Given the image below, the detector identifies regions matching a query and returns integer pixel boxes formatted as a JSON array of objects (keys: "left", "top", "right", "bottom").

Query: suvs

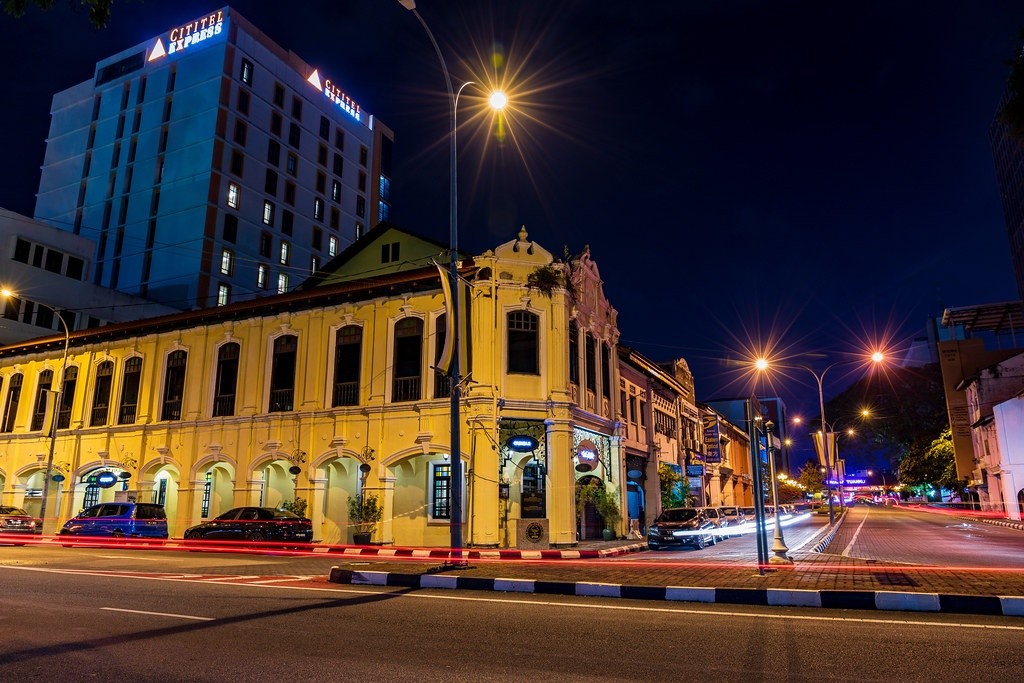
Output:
[{"left": 647, "top": 507, "right": 717, "bottom": 551}]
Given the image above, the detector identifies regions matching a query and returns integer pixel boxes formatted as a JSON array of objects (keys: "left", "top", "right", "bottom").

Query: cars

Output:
[
  {"left": 697, "top": 506, "right": 731, "bottom": 541},
  {"left": 0, "top": 505, "right": 36, "bottom": 546},
  {"left": 183, "top": 506, "right": 313, "bottom": 554}
]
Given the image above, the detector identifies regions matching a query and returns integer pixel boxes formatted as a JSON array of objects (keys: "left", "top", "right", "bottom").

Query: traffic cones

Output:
[{"left": 741, "top": 503, "right": 798, "bottom": 525}]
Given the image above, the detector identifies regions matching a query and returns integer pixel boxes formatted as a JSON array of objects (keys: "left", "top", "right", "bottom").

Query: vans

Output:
[
  {"left": 718, "top": 505, "right": 749, "bottom": 537},
  {"left": 56, "top": 501, "right": 169, "bottom": 549}
]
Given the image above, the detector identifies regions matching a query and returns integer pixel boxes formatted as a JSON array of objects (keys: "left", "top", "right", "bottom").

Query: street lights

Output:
[
  {"left": 834, "top": 427, "right": 855, "bottom": 514},
  {"left": 793, "top": 409, "right": 870, "bottom": 432},
  {"left": 0, "top": 287, "right": 69, "bottom": 518},
  {"left": 783, "top": 437, "right": 791, "bottom": 476},
  {"left": 753, "top": 347, "right": 885, "bottom": 526},
  {"left": 400, "top": 0, "right": 511, "bottom": 556}
]
